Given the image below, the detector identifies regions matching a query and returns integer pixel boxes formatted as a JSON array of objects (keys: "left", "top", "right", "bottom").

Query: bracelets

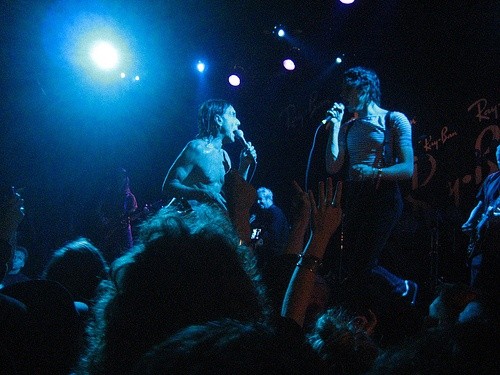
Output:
[
  {"left": 296, "top": 253, "right": 323, "bottom": 274},
  {"left": 372, "top": 166, "right": 375, "bottom": 177},
  {"left": 377, "top": 167, "right": 383, "bottom": 179}
]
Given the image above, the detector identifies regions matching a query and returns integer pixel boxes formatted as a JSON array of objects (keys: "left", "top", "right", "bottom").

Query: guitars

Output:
[
  {"left": 85, "top": 201, "right": 151, "bottom": 244},
  {"left": 467, "top": 195, "right": 500, "bottom": 259}
]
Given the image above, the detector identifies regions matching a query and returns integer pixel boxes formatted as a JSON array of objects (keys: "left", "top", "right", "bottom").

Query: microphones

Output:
[
  {"left": 235, "top": 130, "right": 257, "bottom": 164},
  {"left": 322, "top": 102, "right": 345, "bottom": 125}
]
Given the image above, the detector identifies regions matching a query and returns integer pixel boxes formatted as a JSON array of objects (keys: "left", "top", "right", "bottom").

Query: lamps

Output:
[
  {"left": 282, "top": 46, "right": 301, "bottom": 70},
  {"left": 228, "top": 63, "right": 249, "bottom": 87},
  {"left": 272, "top": 23, "right": 288, "bottom": 40}
]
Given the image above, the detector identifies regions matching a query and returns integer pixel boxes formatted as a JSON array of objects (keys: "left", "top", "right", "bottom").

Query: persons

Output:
[
  {"left": 161, "top": 97, "right": 259, "bottom": 216},
  {"left": 97, "top": 168, "right": 138, "bottom": 263},
  {"left": 252, "top": 186, "right": 290, "bottom": 257},
  {"left": 460, "top": 145, "right": 500, "bottom": 290},
  {"left": 323, "top": 65, "right": 414, "bottom": 265},
  {"left": 0, "top": 178, "right": 500, "bottom": 375}
]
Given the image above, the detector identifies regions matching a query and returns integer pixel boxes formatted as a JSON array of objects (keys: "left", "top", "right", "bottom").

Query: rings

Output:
[
  {"left": 331, "top": 201, "right": 341, "bottom": 208},
  {"left": 325, "top": 199, "right": 330, "bottom": 206}
]
